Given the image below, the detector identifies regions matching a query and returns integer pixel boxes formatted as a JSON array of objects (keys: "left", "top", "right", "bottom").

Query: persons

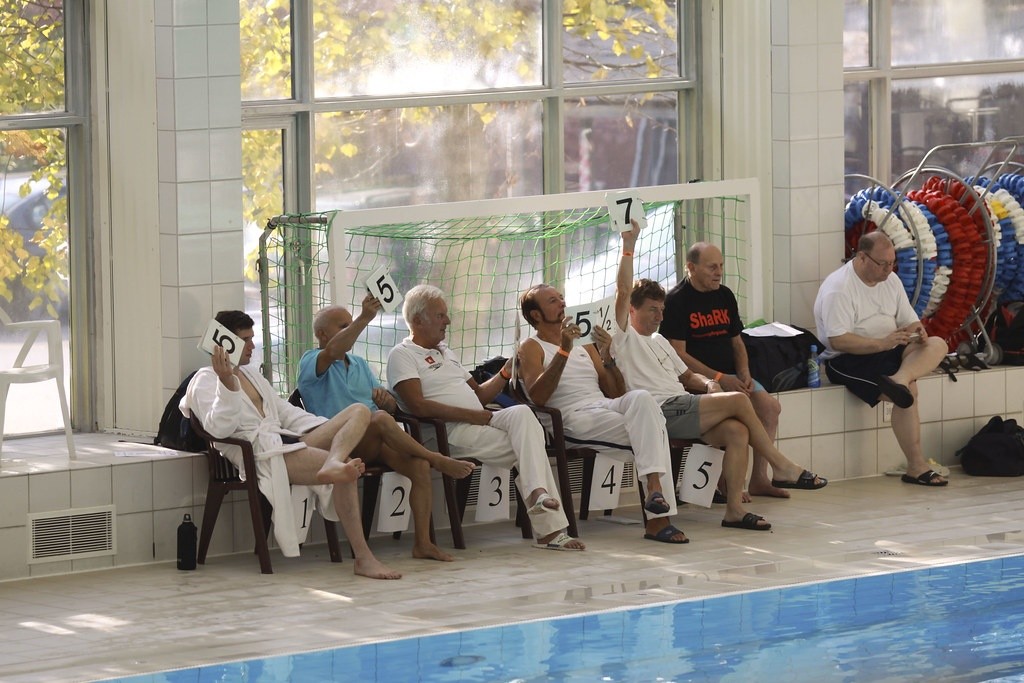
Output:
[
  {"left": 188, "top": 310, "right": 403, "bottom": 580},
  {"left": 659, "top": 242, "right": 791, "bottom": 503},
  {"left": 386, "top": 285, "right": 586, "bottom": 551},
  {"left": 608, "top": 219, "right": 827, "bottom": 531},
  {"left": 297, "top": 292, "right": 476, "bottom": 562},
  {"left": 518, "top": 284, "right": 689, "bottom": 544},
  {"left": 814, "top": 231, "right": 948, "bottom": 485}
]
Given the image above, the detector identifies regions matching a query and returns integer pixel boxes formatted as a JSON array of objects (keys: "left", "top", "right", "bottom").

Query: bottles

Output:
[
  {"left": 807, "top": 345, "right": 820, "bottom": 388},
  {"left": 177, "top": 514, "right": 197, "bottom": 570}
]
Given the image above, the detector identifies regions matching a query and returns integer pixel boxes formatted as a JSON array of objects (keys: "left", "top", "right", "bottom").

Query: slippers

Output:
[
  {"left": 772, "top": 469, "right": 828, "bottom": 490},
  {"left": 721, "top": 512, "right": 771, "bottom": 531},
  {"left": 878, "top": 376, "right": 914, "bottom": 408},
  {"left": 644, "top": 491, "right": 670, "bottom": 515},
  {"left": 901, "top": 470, "right": 948, "bottom": 487},
  {"left": 527, "top": 492, "right": 560, "bottom": 515},
  {"left": 710, "top": 482, "right": 728, "bottom": 504},
  {"left": 643, "top": 523, "right": 689, "bottom": 544},
  {"left": 532, "top": 532, "right": 583, "bottom": 551}
]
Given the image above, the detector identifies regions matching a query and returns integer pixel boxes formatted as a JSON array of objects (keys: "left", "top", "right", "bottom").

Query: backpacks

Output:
[{"left": 154, "top": 370, "right": 209, "bottom": 453}]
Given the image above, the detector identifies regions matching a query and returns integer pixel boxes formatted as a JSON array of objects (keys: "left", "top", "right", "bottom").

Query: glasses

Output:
[{"left": 863, "top": 251, "right": 896, "bottom": 270}]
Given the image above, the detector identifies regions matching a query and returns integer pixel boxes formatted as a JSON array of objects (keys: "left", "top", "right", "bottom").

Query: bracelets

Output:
[
  {"left": 715, "top": 372, "right": 722, "bottom": 380},
  {"left": 623, "top": 250, "right": 634, "bottom": 256},
  {"left": 557, "top": 345, "right": 569, "bottom": 358},
  {"left": 500, "top": 366, "right": 510, "bottom": 380},
  {"left": 705, "top": 380, "right": 717, "bottom": 387},
  {"left": 603, "top": 357, "right": 616, "bottom": 369}
]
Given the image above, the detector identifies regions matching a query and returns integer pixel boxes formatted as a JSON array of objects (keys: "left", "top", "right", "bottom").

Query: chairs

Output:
[
  {"left": 0, "top": 307, "right": 76, "bottom": 457},
  {"left": 188, "top": 407, "right": 343, "bottom": 574},
  {"left": 508, "top": 379, "right": 648, "bottom": 538},
  {"left": 670, "top": 437, "right": 727, "bottom": 506},
  {"left": 385, "top": 389, "right": 534, "bottom": 549},
  {"left": 299, "top": 396, "right": 437, "bottom": 560}
]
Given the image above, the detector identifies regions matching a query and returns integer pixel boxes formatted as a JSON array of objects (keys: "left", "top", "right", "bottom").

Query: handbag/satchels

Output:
[
  {"left": 468, "top": 354, "right": 525, "bottom": 405},
  {"left": 743, "top": 321, "right": 827, "bottom": 392},
  {"left": 956, "top": 416, "right": 1024, "bottom": 477}
]
